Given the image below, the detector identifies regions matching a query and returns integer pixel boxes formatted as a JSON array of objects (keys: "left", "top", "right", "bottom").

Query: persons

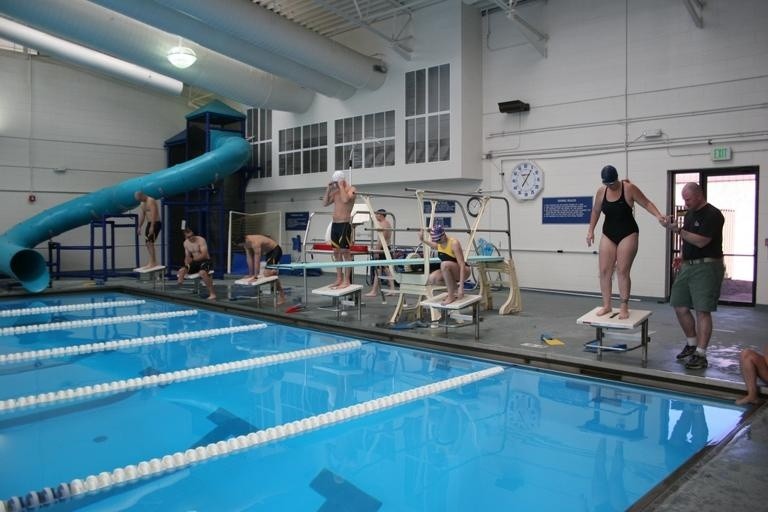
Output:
[
  {"left": 363, "top": 210, "right": 395, "bottom": 297},
  {"left": 417, "top": 225, "right": 471, "bottom": 305},
  {"left": 232, "top": 233, "right": 289, "bottom": 306},
  {"left": 175, "top": 228, "right": 216, "bottom": 300},
  {"left": 134, "top": 191, "right": 165, "bottom": 269},
  {"left": 732, "top": 347, "right": 768, "bottom": 406},
  {"left": 321, "top": 171, "right": 357, "bottom": 290},
  {"left": 127, "top": 294, "right": 711, "bottom": 511},
  {"left": 585, "top": 165, "right": 675, "bottom": 321},
  {"left": 659, "top": 182, "right": 727, "bottom": 369}
]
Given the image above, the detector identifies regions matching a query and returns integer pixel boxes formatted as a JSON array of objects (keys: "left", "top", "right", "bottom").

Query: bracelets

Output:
[{"left": 677, "top": 227, "right": 683, "bottom": 235}]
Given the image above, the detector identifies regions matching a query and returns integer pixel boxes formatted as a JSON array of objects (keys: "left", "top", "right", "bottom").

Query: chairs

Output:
[{"left": 326, "top": 201, "right": 371, "bottom": 246}]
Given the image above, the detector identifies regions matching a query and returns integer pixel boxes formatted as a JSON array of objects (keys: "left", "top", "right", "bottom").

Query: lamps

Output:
[{"left": 166, "top": 31, "right": 200, "bottom": 70}]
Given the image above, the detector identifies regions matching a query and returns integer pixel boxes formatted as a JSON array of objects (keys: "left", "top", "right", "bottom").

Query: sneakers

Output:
[
  {"left": 676, "top": 344, "right": 697, "bottom": 359},
  {"left": 685, "top": 352, "right": 708, "bottom": 370}
]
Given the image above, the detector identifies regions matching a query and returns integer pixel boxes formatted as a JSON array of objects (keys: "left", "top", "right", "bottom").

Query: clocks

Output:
[{"left": 507, "top": 160, "right": 546, "bottom": 202}]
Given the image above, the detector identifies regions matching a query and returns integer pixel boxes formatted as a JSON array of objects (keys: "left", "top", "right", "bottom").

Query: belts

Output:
[{"left": 686, "top": 258, "right": 721, "bottom": 265}]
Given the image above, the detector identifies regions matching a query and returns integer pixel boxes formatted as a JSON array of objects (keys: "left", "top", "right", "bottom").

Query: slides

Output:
[{"left": 0, "top": 129, "right": 251, "bottom": 294}]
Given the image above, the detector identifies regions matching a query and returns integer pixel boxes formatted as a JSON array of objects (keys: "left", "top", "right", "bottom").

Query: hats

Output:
[
  {"left": 430, "top": 225, "right": 445, "bottom": 242},
  {"left": 601, "top": 165, "right": 618, "bottom": 182},
  {"left": 332, "top": 171, "right": 346, "bottom": 182}
]
